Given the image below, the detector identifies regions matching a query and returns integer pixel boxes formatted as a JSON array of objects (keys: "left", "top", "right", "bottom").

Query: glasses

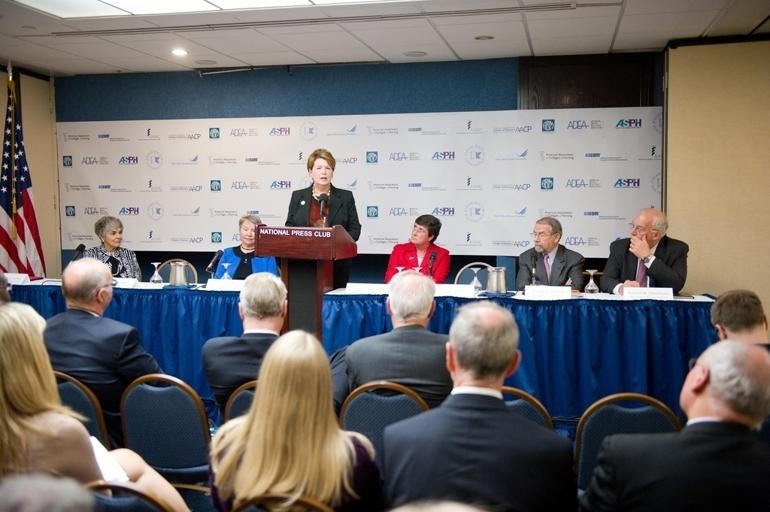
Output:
[
  {"left": 104, "top": 279, "right": 117, "bottom": 288},
  {"left": 530, "top": 232, "right": 553, "bottom": 238}
]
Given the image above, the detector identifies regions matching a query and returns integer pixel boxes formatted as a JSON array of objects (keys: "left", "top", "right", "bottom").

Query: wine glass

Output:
[
  {"left": 220, "top": 263, "right": 233, "bottom": 280},
  {"left": 584, "top": 269, "right": 600, "bottom": 294},
  {"left": 394, "top": 267, "right": 423, "bottom": 273},
  {"left": 469, "top": 267, "right": 482, "bottom": 292},
  {"left": 149, "top": 263, "right": 164, "bottom": 287}
]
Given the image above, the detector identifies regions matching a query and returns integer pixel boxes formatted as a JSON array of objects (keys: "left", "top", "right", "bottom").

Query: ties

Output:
[
  {"left": 543, "top": 253, "right": 551, "bottom": 283},
  {"left": 636, "top": 258, "right": 646, "bottom": 288}
]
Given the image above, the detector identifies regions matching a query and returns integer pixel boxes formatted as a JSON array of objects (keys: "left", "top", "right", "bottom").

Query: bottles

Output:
[
  {"left": 170, "top": 261, "right": 190, "bottom": 287},
  {"left": 486, "top": 267, "right": 507, "bottom": 293}
]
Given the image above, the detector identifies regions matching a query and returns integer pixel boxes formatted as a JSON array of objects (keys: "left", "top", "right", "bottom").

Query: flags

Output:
[{"left": 0, "top": 67, "right": 47, "bottom": 282}]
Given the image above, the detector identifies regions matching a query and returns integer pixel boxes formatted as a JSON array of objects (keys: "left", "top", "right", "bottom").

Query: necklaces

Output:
[
  {"left": 311, "top": 186, "right": 332, "bottom": 201},
  {"left": 240, "top": 244, "right": 256, "bottom": 251}
]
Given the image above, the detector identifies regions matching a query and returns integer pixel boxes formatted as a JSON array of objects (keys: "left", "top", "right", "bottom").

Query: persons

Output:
[
  {"left": 79, "top": 217, "right": 142, "bottom": 282},
  {"left": 213, "top": 215, "right": 281, "bottom": 278},
  {"left": 374, "top": 300, "right": 578, "bottom": 512},
  {"left": 1, "top": 258, "right": 451, "bottom": 512},
  {"left": 577, "top": 338, "right": 768, "bottom": 512},
  {"left": 710, "top": 289, "right": 769, "bottom": 351},
  {"left": 284, "top": 149, "right": 361, "bottom": 289},
  {"left": 515, "top": 217, "right": 586, "bottom": 293},
  {"left": 384, "top": 215, "right": 450, "bottom": 284},
  {"left": 600, "top": 208, "right": 689, "bottom": 298}
]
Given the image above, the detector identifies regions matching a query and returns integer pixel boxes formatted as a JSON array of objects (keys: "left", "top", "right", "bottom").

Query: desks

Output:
[
  {"left": 9, "top": 277, "right": 243, "bottom": 428},
  {"left": 322, "top": 281, "right": 719, "bottom": 420}
]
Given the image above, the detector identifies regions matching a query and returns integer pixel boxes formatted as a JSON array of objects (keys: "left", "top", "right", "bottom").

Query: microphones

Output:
[
  {"left": 68, "top": 244, "right": 86, "bottom": 262},
  {"left": 530, "top": 255, "right": 537, "bottom": 281},
  {"left": 205, "top": 249, "right": 224, "bottom": 272},
  {"left": 319, "top": 193, "right": 330, "bottom": 226},
  {"left": 428, "top": 251, "right": 437, "bottom": 278}
]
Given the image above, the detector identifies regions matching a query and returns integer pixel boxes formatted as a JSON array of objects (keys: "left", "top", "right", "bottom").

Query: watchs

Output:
[{"left": 643, "top": 254, "right": 652, "bottom": 264}]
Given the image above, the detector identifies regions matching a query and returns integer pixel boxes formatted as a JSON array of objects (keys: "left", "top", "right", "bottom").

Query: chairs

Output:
[
  {"left": 502, "top": 385, "right": 553, "bottom": 430},
  {"left": 119, "top": 373, "right": 212, "bottom": 490},
  {"left": 230, "top": 493, "right": 334, "bottom": 512},
  {"left": 575, "top": 393, "right": 680, "bottom": 491},
  {"left": 339, "top": 379, "right": 429, "bottom": 492},
  {"left": 172, "top": 483, "right": 217, "bottom": 512},
  {"left": 225, "top": 380, "right": 259, "bottom": 423},
  {"left": 53, "top": 370, "right": 110, "bottom": 452},
  {"left": 82, "top": 480, "right": 175, "bottom": 512},
  {"left": 149, "top": 259, "right": 198, "bottom": 283},
  {"left": 454, "top": 261, "right": 493, "bottom": 289}
]
